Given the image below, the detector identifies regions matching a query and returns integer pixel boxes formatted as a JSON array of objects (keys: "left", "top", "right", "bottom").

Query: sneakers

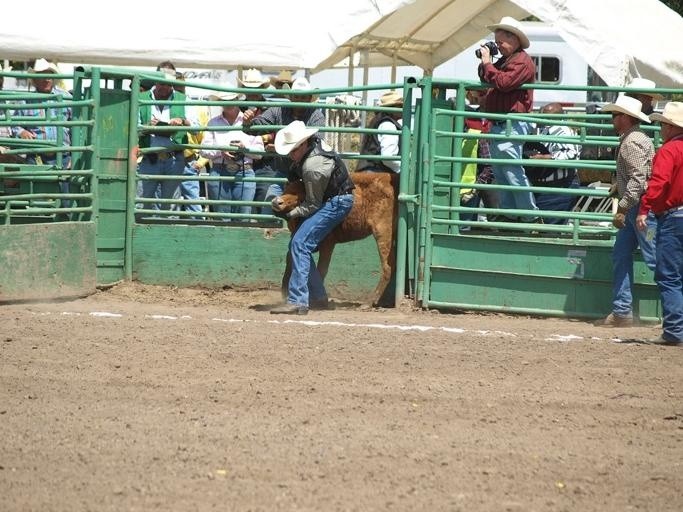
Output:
[{"left": 459, "top": 219, "right": 470, "bottom": 232}]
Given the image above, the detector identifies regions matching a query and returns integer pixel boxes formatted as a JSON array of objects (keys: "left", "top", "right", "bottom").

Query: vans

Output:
[{"left": 487, "top": 18, "right": 589, "bottom": 119}]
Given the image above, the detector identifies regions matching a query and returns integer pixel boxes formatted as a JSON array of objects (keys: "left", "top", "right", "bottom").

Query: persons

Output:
[
  {"left": 594, "top": 93, "right": 655, "bottom": 329},
  {"left": 624, "top": 78, "right": 666, "bottom": 139},
  {"left": 136, "top": 60, "right": 405, "bottom": 223},
  {"left": 478, "top": 16, "right": 545, "bottom": 234},
  {"left": 11, "top": 59, "right": 73, "bottom": 220},
  {"left": 271, "top": 120, "right": 356, "bottom": 314},
  {"left": 460, "top": 87, "right": 582, "bottom": 236},
  {"left": 636, "top": 101, "right": 683, "bottom": 346}
]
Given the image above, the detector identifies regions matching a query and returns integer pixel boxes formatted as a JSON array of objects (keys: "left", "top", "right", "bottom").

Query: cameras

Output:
[
  {"left": 475, "top": 42, "right": 498, "bottom": 58},
  {"left": 229, "top": 142, "right": 244, "bottom": 160}
]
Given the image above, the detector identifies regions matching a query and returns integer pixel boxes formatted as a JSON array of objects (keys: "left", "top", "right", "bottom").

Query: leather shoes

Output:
[
  {"left": 309, "top": 299, "right": 328, "bottom": 308},
  {"left": 270, "top": 303, "right": 307, "bottom": 315},
  {"left": 594, "top": 312, "right": 633, "bottom": 327},
  {"left": 648, "top": 333, "right": 683, "bottom": 346}
]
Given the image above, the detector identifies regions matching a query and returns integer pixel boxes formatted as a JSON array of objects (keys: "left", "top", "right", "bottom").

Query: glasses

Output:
[
  {"left": 612, "top": 113, "right": 624, "bottom": 120},
  {"left": 624, "top": 93, "right": 641, "bottom": 98}
]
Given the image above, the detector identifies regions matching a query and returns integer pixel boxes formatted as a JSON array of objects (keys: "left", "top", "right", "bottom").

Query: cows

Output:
[{"left": 271, "top": 171, "right": 400, "bottom": 310}]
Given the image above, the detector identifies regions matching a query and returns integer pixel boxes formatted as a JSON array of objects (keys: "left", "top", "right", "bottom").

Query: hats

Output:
[
  {"left": 274, "top": 120, "right": 320, "bottom": 155},
  {"left": 600, "top": 96, "right": 651, "bottom": 124},
  {"left": 448, "top": 97, "right": 480, "bottom": 110},
  {"left": 0, "top": 64, "right": 13, "bottom": 73},
  {"left": 207, "top": 69, "right": 320, "bottom": 102},
  {"left": 649, "top": 102, "right": 683, "bottom": 128},
  {"left": 624, "top": 78, "right": 664, "bottom": 100},
  {"left": 28, "top": 57, "right": 61, "bottom": 87},
  {"left": 379, "top": 92, "right": 403, "bottom": 107},
  {"left": 487, "top": 17, "right": 530, "bottom": 49}
]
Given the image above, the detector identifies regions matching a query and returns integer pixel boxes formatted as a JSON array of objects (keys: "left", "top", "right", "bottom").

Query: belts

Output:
[
  {"left": 156, "top": 150, "right": 182, "bottom": 160},
  {"left": 27, "top": 152, "right": 70, "bottom": 160},
  {"left": 492, "top": 119, "right": 506, "bottom": 126},
  {"left": 213, "top": 163, "right": 254, "bottom": 172},
  {"left": 655, "top": 205, "right": 683, "bottom": 219}
]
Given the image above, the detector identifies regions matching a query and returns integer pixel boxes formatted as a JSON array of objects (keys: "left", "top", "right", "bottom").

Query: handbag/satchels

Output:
[{"left": 523, "top": 142, "right": 553, "bottom": 179}]
